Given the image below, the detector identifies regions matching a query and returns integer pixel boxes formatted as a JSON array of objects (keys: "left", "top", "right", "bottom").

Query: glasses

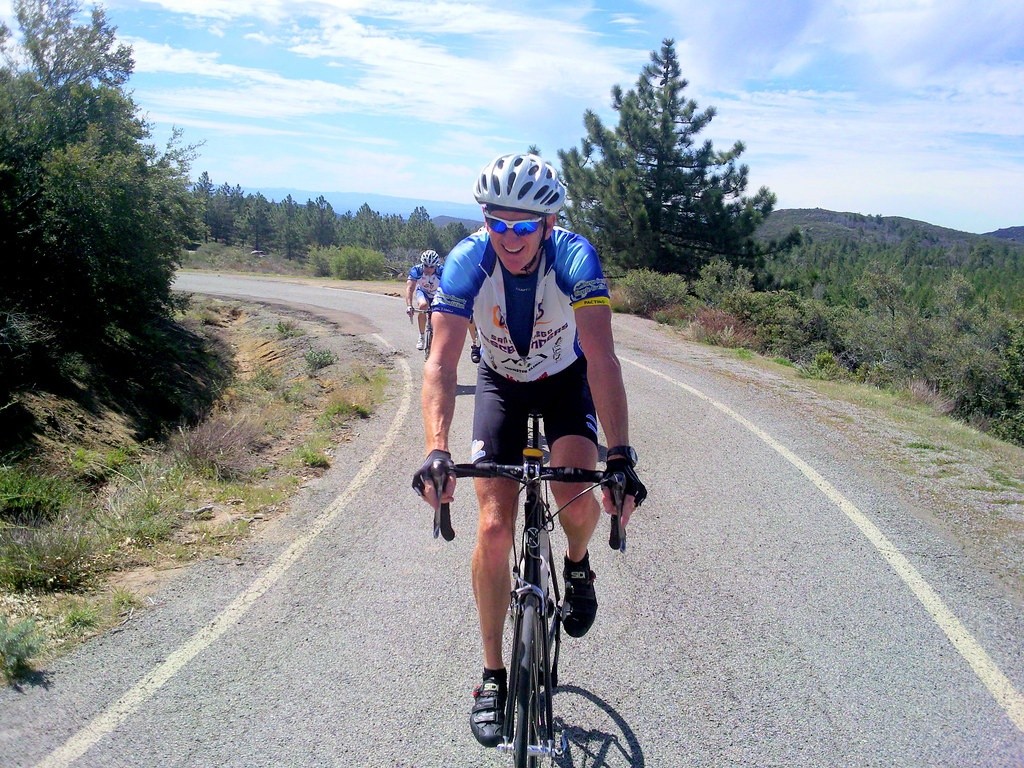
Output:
[
  {"left": 424, "top": 264, "right": 435, "bottom": 268},
  {"left": 484, "top": 206, "right": 545, "bottom": 237}
]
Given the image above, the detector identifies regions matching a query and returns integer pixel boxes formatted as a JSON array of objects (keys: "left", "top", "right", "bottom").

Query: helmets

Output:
[
  {"left": 420, "top": 250, "right": 440, "bottom": 267},
  {"left": 473, "top": 153, "right": 565, "bottom": 216}
]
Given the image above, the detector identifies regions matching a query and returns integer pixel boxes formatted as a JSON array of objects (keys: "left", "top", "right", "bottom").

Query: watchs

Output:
[{"left": 607, "top": 445, "right": 638, "bottom": 468}]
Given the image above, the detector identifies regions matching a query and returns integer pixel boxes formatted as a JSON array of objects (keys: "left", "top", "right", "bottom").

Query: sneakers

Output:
[
  {"left": 561, "top": 557, "right": 598, "bottom": 637},
  {"left": 471, "top": 345, "right": 481, "bottom": 363},
  {"left": 416, "top": 335, "right": 425, "bottom": 349},
  {"left": 470, "top": 677, "right": 506, "bottom": 747}
]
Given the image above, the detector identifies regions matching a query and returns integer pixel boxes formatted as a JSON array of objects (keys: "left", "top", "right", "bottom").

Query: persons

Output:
[
  {"left": 469, "top": 306, "right": 481, "bottom": 364},
  {"left": 406, "top": 250, "right": 444, "bottom": 350},
  {"left": 411, "top": 154, "right": 648, "bottom": 749}
]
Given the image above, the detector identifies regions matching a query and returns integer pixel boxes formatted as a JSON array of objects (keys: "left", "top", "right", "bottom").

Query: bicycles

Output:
[
  {"left": 408, "top": 305, "right": 433, "bottom": 363},
  {"left": 430, "top": 413, "right": 625, "bottom": 767}
]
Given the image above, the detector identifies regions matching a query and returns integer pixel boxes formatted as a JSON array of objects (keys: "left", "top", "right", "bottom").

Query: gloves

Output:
[{"left": 406, "top": 306, "right": 414, "bottom": 317}]
